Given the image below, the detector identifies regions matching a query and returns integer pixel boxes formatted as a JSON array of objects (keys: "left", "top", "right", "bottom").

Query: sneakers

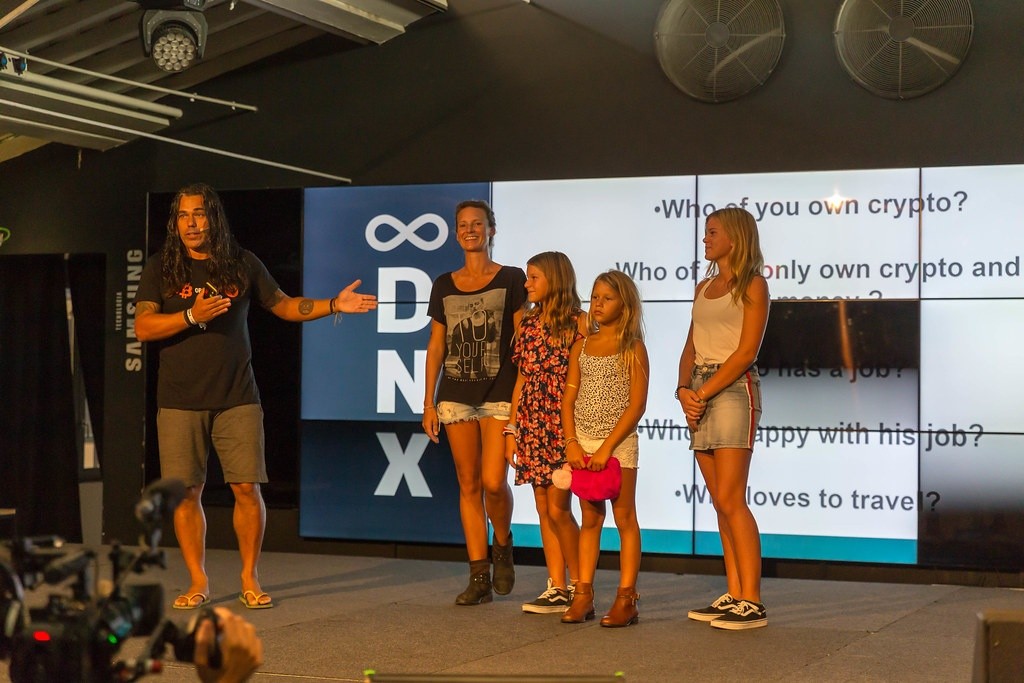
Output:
[
  {"left": 567, "top": 584, "right": 576, "bottom": 602},
  {"left": 710, "top": 600, "right": 767, "bottom": 630},
  {"left": 688, "top": 593, "right": 742, "bottom": 621},
  {"left": 521, "top": 578, "right": 572, "bottom": 614}
]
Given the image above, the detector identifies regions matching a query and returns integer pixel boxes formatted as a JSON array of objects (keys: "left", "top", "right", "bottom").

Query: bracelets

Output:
[
  {"left": 329, "top": 297, "right": 342, "bottom": 325},
  {"left": 564, "top": 437, "right": 579, "bottom": 446},
  {"left": 695, "top": 393, "right": 705, "bottom": 404},
  {"left": 183, "top": 309, "right": 198, "bottom": 328},
  {"left": 503, "top": 424, "right": 518, "bottom": 436},
  {"left": 424, "top": 406, "right": 435, "bottom": 410}
]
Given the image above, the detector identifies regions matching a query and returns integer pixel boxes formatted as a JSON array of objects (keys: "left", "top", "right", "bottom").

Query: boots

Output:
[
  {"left": 600, "top": 587, "right": 640, "bottom": 627},
  {"left": 492, "top": 530, "right": 515, "bottom": 595},
  {"left": 456, "top": 559, "right": 493, "bottom": 605},
  {"left": 561, "top": 583, "right": 596, "bottom": 622}
]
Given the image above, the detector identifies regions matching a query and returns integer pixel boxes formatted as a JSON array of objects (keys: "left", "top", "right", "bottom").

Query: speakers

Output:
[{"left": 970, "top": 610, "right": 1024, "bottom": 683}]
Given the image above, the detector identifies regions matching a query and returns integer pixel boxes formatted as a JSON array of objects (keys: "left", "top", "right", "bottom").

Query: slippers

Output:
[
  {"left": 173, "top": 593, "right": 211, "bottom": 609},
  {"left": 239, "top": 590, "right": 273, "bottom": 608}
]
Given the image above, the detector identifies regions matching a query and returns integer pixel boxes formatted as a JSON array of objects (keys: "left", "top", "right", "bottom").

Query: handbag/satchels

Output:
[{"left": 552, "top": 456, "right": 622, "bottom": 502}]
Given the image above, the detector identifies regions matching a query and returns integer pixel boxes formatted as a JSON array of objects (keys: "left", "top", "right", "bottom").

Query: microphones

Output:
[{"left": 133, "top": 476, "right": 186, "bottom": 523}]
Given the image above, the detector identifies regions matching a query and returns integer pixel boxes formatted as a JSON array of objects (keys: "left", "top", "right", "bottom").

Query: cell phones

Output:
[{"left": 204, "top": 282, "right": 217, "bottom": 300}]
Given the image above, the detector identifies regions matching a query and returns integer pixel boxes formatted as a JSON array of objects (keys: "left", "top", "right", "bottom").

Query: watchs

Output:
[{"left": 674, "top": 385, "right": 687, "bottom": 400}]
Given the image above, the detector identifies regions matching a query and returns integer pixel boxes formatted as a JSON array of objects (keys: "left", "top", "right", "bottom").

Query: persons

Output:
[
  {"left": 7, "top": 592, "right": 265, "bottom": 683},
  {"left": 132, "top": 181, "right": 380, "bottom": 610},
  {"left": 678, "top": 208, "right": 771, "bottom": 631},
  {"left": 504, "top": 251, "right": 590, "bottom": 613},
  {"left": 422, "top": 201, "right": 529, "bottom": 605},
  {"left": 560, "top": 269, "right": 650, "bottom": 625}
]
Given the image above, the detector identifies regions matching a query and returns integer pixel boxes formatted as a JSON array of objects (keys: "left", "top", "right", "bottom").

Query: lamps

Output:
[{"left": 139, "top": 9, "right": 208, "bottom": 74}]
